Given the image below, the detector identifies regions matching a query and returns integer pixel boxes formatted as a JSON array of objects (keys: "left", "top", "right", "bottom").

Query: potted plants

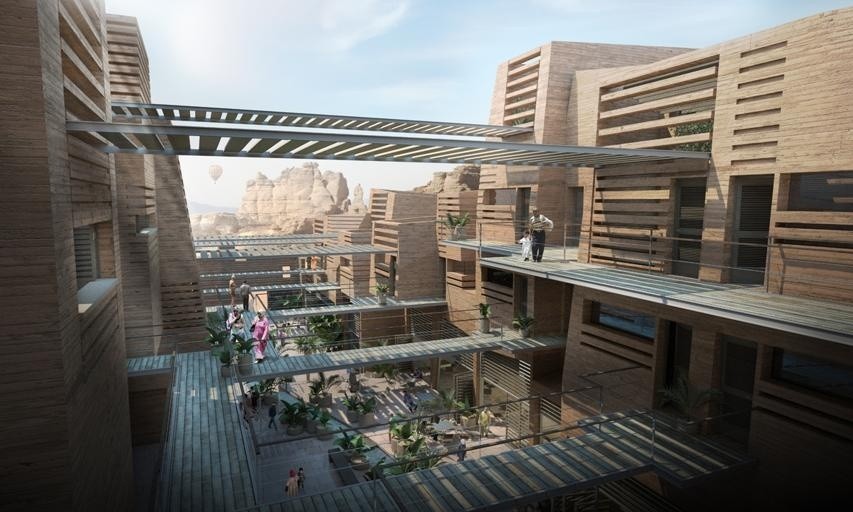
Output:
[
  {"left": 432, "top": 212, "right": 470, "bottom": 241},
  {"left": 204, "top": 282, "right": 536, "bottom": 477},
  {"left": 653, "top": 377, "right": 724, "bottom": 434}
]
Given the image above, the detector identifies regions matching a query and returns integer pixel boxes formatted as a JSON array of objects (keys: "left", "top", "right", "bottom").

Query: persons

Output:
[
  {"left": 413, "top": 368, "right": 423, "bottom": 379},
  {"left": 227, "top": 305, "right": 246, "bottom": 359},
  {"left": 284, "top": 469, "right": 298, "bottom": 497},
  {"left": 229, "top": 275, "right": 237, "bottom": 308},
  {"left": 515, "top": 230, "right": 532, "bottom": 261},
  {"left": 528, "top": 207, "right": 553, "bottom": 262},
  {"left": 479, "top": 407, "right": 489, "bottom": 437},
  {"left": 246, "top": 386, "right": 260, "bottom": 415},
  {"left": 403, "top": 390, "right": 416, "bottom": 410},
  {"left": 240, "top": 279, "right": 254, "bottom": 311},
  {"left": 278, "top": 330, "right": 287, "bottom": 345},
  {"left": 417, "top": 420, "right": 428, "bottom": 434},
  {"left": 267, "top": 402, "right": 280, "bottom": 430},
  {"left": 297, "top": 468, "right": 304, "bottom": 488},
  {"left": 456, "top": 439, "right": 466, "bottom": 462},
  {"left": 252, "top": 309, "right": 270, "bottom": 364}
]
